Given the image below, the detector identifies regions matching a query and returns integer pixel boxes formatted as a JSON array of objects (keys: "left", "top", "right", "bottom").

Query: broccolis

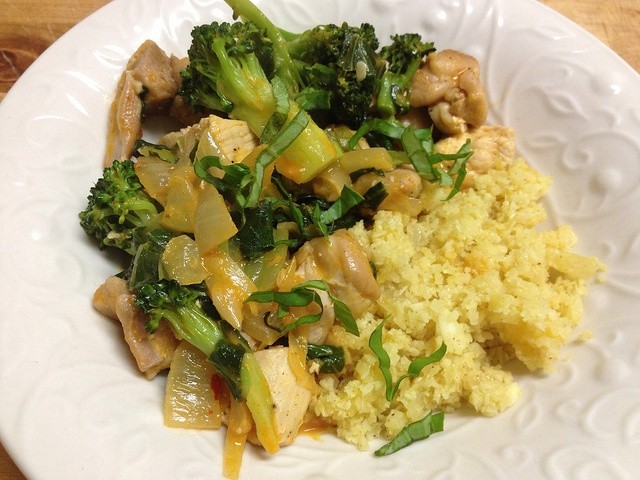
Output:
[{"left": 79, "top": 1, "right": 435, "bottom": 450}]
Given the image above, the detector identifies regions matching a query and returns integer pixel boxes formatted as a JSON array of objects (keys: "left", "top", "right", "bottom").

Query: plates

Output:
[{"left": 0, "top": 0, "right": 640, "bottom": 480}]
